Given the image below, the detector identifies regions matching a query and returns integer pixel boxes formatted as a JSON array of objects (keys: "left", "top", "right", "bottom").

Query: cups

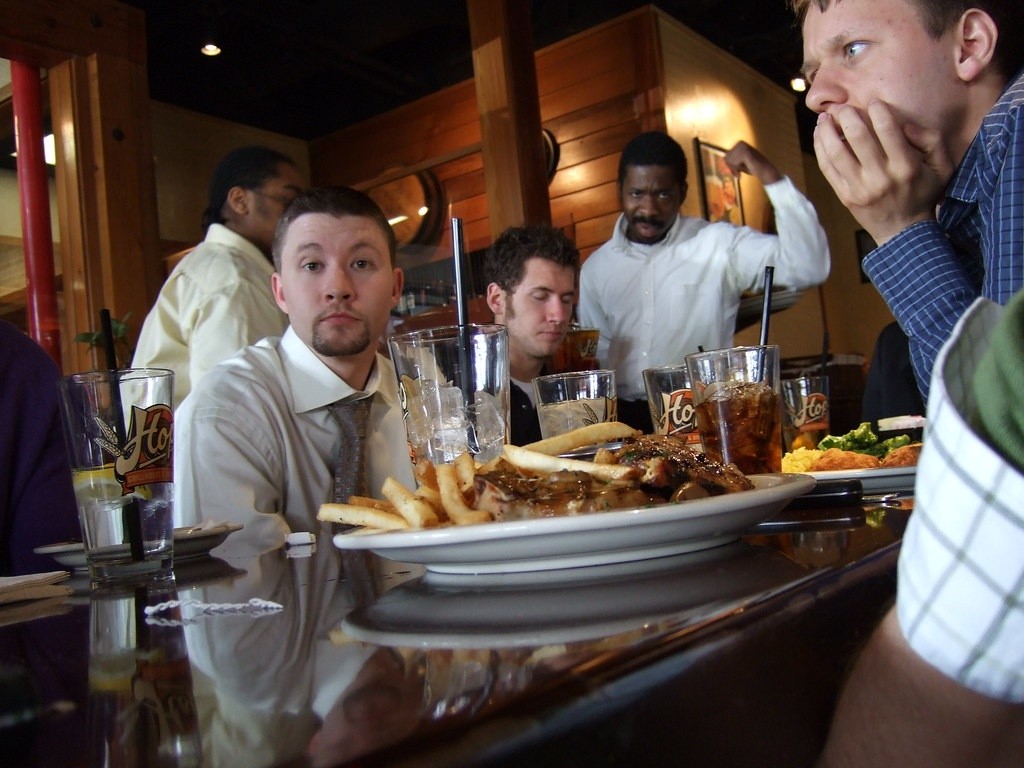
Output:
[
  {"left": 562, "top": 322, "right": 600, "bottom": 372},
  {"left": 685, "top": 344, "right": 783, "bottom": 474},
  {"left": 781, "top": 376, "right": 829, "bottom": 450},
  {"left": 531, "top": 370, "right": 617, "bottom": 439},
  {"left": 387, "top": 324, "right": 511, "bottom": 492},
  {"left": 643, "top": 365, "right": 705, "bottom": 452},
  {"left": 58, "top": 366, "right": 175, "bottom": 582}
]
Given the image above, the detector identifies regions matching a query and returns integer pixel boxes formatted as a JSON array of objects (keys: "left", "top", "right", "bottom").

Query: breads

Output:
[
  {"left": 880, "top": 442, "right": 922, "bottom": 467},
  {"left": 807, "top": 448, "right": 880, "bottom": 472}
]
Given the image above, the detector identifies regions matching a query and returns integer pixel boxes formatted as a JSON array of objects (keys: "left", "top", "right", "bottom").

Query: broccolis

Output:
[{"left": 817, "top": 421, "right": 909, "bottom": 458}]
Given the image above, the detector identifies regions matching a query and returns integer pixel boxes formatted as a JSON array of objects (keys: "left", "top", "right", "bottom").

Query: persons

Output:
[
  {"left": 0, "top": 319, "right": 139, "bottom": 768},
  {"left": 124, "top": 141, "right": 484, "bottom": 536},
  {"left": 468, "top": 224, "right": 637, "bottom": 461},
  {"left": 576, "top": 130, "right": 832, "bottom": 436},
  {"left": 795, "top": 0, "right": 1024, "bottom": 414},
  {"left": 173, "top": 559, "right": 494, "bottom": 768},
  {"left": 822, "top": 288, "right": 1024, "bottom": 768}
]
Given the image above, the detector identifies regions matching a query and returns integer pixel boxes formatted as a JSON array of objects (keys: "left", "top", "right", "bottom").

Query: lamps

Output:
[{"left": 197, "top": 26, "right": 222, "bottom": 58}]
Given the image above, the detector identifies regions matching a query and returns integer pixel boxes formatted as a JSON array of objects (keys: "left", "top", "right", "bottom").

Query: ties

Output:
[
  {"left": 323, "top": 391, "right": 381, "bottom": 532},
  {"left": 333, "top": 534, "right": 380, "bottom": 610}
]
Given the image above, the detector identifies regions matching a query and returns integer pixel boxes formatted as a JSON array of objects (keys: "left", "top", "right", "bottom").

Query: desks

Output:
[{"left": 0, "top": 495, "right": 913, "bottom": 768}]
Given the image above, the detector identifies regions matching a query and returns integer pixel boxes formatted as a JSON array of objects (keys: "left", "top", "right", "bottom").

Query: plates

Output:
[
  {"left": 344, "top": 535, "right": 807, "bottom": 648},
  {"left": 34, "top": 521, "right": 244, "bottom": 570},
  {"left": 805, "top": 466, "right": 916, "bottom": 492},
  {"left": 331, "top": 469, "right": 817, "bottom": 570}
]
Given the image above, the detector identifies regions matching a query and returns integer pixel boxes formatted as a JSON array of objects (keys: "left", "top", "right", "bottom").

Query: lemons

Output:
[{"left": 72, "top": 464, "right": 152, "bottom": 502}]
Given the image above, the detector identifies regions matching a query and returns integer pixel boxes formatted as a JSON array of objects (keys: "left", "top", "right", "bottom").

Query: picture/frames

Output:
[
  {"left": 694, "top": 139, "right": 749, "bottom": 229},
  {"left": 854, "top": 230, "right": 877, "bottom": 284}
]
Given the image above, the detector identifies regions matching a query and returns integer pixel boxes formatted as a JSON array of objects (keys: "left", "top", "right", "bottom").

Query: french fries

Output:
[{"left": 316, "top": 421, "right": 641, "bottom": 528}]
